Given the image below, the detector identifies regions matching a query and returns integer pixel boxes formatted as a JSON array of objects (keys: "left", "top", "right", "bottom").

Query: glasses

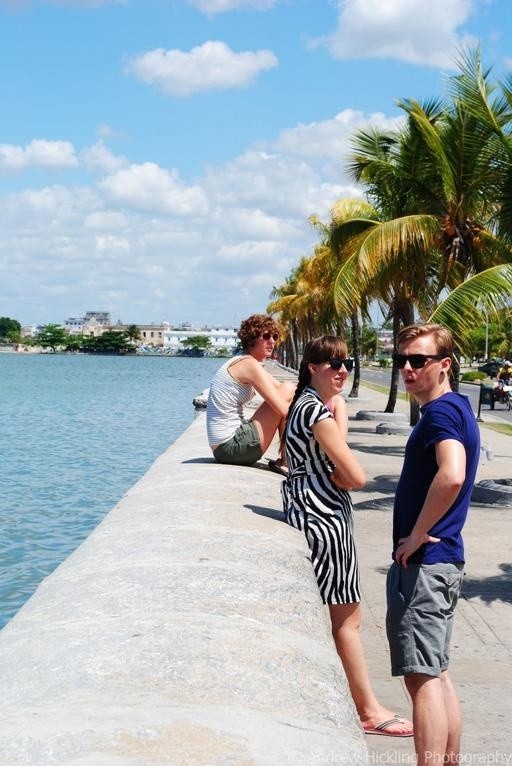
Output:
[
  {"left": 263, "top": 333, "right": 278, "bottom": 341},
  {"left": 328, "top": 358, "right": 353, "bottom": 372},
  {"left": 391, "top": 354, "right": 444, "bottom": 368}
]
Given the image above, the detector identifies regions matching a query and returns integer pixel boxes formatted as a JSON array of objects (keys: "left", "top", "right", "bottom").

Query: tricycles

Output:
[{"left": 479, "top": 378, "right": 512, "bottom": 411}]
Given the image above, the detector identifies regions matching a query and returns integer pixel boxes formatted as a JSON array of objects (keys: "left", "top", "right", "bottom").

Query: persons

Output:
[
  {"left": 206, "top": 315, "right": 297, "bottom": 477},
  {"left": 497, "top": 361, "right": 512, "bottom": 404},
  {"left": 387, "top": 325, "right": 480, "bottom": 766},
  {"left": 281, "top": 335, "right": 413, "bottom": 737}
]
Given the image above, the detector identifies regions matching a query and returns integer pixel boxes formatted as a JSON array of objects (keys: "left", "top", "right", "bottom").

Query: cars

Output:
[{"left": 478, "top": 356, "right": 512, "bottom": 379}]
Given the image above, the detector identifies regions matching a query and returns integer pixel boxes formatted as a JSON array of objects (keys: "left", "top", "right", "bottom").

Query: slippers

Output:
[
  {"left": 269, "top": 457, "right": 289, "bottom": 477},
  {"left": 365, "top": 714, "right": 415, "bottom": 737}
]
